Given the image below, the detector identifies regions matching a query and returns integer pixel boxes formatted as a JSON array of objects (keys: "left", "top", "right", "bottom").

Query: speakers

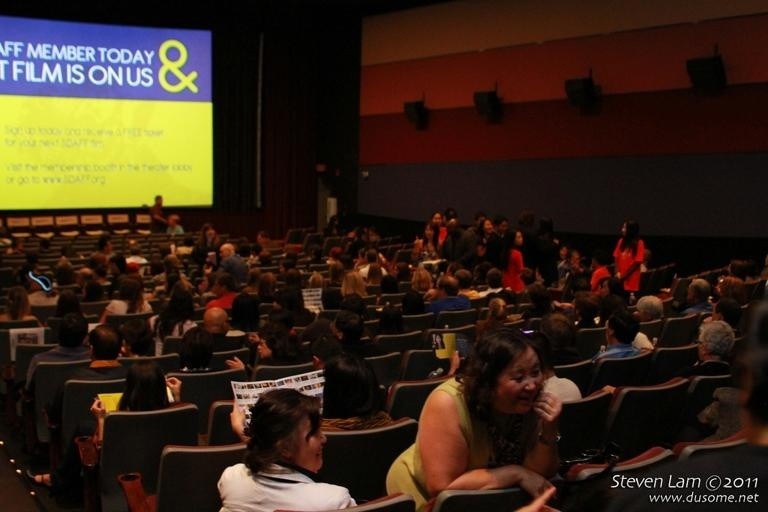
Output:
[
  {"left": 404, "top": 101, "right": 424, "bottom": 122},
  {"left": 686, "top": 55, "right": 728, "bottom": 89},
  {"left": 472, "top": 90, "right": 500, "bottom": 113},
  {"left": 564, "top": 77, "right": 596, "bottom": 105}
]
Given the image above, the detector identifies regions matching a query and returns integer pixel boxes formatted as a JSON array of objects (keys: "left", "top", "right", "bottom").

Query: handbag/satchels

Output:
[{"left": 558, "top": 439, "right": 625, "bottom": 477}]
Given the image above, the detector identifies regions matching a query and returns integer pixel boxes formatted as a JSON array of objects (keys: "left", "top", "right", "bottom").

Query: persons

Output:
[{"left": 0, "top": 194, "right": 767, "bottom": 512}]
